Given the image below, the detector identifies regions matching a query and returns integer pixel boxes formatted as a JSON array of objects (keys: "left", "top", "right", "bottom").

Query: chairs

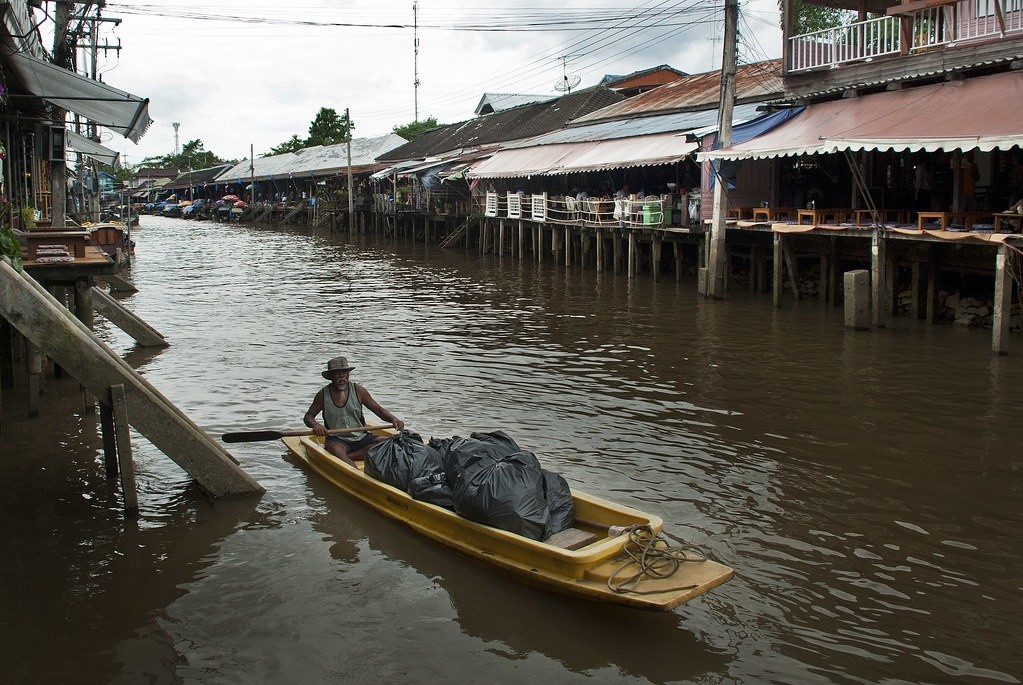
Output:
[{"left": 520, "top": 192, "right": 669, "bottom": 225}]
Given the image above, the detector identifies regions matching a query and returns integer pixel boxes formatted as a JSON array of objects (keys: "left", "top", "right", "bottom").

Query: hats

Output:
[{"left": 322, "top": 357, "right": 355, "bottom": 380}]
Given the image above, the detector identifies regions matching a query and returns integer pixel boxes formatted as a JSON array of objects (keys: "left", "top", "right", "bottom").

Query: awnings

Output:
[
  {"left": 64, "top": 125, "right": 120, "bottom": 168},
  {"left": 368, "top": 130, "right": 701, "bottom": 206},
  {"left": 6, "top": 46, "right": 153, "bottom": 144},
  {"left": 694, "top": 68, "right": 1022, "bottom": 224}
]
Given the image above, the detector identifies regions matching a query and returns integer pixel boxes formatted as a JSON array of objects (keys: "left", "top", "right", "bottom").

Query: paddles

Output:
[{"left": 223, "top": 424, "right": 396, "bottom": 444}]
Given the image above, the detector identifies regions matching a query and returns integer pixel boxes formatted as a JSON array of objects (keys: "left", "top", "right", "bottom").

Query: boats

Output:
[{"left": 279, "top": 426, "right": 735, "bottom": 611}]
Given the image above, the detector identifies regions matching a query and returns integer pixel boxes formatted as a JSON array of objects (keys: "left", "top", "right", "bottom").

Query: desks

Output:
[
  {"left": 752, "top": 207, "right": 793, "bottom": 222},
  {"left": 728, "top": 208, "right": 754, "bottom": 220},
  {"left": 992, "top": 213, "right": 1023, "bottom": 233},
  {"left": 917, "top": 211, "right": 971, "bottom": 232},
  {"left": 796, "top": 208, "right": 841, "bottom": 227},
  {"left": 854, "top": 209, "right": 901, "bottom": 226}
]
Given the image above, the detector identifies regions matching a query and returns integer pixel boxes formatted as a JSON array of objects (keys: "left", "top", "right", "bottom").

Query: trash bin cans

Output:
[{"left": 643, "top": 204, "right": 672, "bottom": 226}]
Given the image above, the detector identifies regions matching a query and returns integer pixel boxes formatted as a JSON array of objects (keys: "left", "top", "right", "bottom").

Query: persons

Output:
[{"left": 302, "top": 355, "right": 404, "bottom": 469}]
